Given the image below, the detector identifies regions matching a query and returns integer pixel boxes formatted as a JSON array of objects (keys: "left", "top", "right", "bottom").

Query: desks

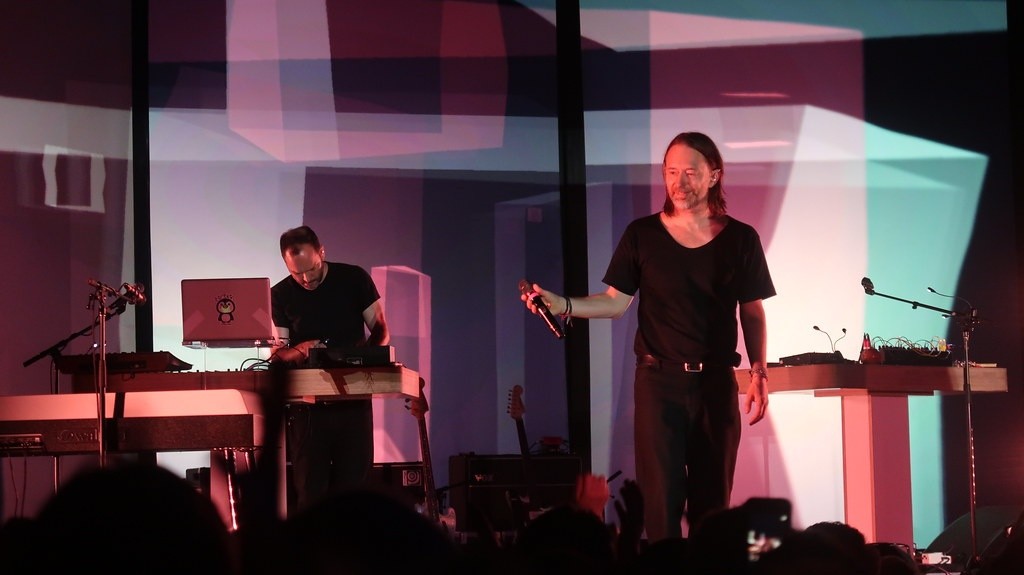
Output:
[
  {"left": 73, "top": 367, "right": 420, "bottom": 532},
  {"left": 735, "top": 366, "right": 1007, "bottom": 555}
]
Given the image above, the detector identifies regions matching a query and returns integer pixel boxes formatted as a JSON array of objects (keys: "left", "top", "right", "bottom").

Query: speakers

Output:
[
  {"left": 372, "top": 462, "right": 427, "bottom": 514},
  {"left": 745, "top": 497, "right": 791, "bottom": 527},
  {"left": 922, "top": 506, "right": 1024, "bottom": 575},
  {"left": 448, "top": 453, "right": 583, "bottom": 533}
]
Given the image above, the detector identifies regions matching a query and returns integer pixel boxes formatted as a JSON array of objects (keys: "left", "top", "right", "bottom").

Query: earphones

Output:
[
  {"left": 710, "top": 173, "right": 717, "bottom": 182},
  {"left": 320, "top": 250, "right": 324, "bottom": 258}
]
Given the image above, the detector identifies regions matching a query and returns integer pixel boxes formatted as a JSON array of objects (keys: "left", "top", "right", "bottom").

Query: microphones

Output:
[
  {"left": 519, "top": 280, "right": 565, "bottom": 339},
  {"left": 106, "top": 283, "right": 144, "bottom": 312},
  {"left": 124, "top": 283, "right": 146, "bottom": 306}
]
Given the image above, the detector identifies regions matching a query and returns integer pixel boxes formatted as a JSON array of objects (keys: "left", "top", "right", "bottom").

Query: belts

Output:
[{"left": 636, "top": 355, "right": 733, "bottom": 373}]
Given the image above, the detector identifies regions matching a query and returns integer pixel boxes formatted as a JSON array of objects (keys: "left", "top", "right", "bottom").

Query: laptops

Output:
[{"left": 181, "top": 278, "right": 290, "bottom": 351}]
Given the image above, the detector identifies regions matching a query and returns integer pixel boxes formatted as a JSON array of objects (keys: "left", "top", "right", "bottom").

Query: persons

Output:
[
  {"left": 0, "top": 454, "right": 924, "bottom": 575},
  {"left": 521, "top": 132, "right": 776, "bottom": 543},
  {"left": 270, "top": 226, "right": 392, "bottom": 526}
]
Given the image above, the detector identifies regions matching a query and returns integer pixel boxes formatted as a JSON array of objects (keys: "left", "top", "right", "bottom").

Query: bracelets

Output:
[
  {"left": 750, "top": 367, "right": 768, "bottom": 382},
  {"left": 559, "top": 295, "right": 573, "bottom": 326}
]
